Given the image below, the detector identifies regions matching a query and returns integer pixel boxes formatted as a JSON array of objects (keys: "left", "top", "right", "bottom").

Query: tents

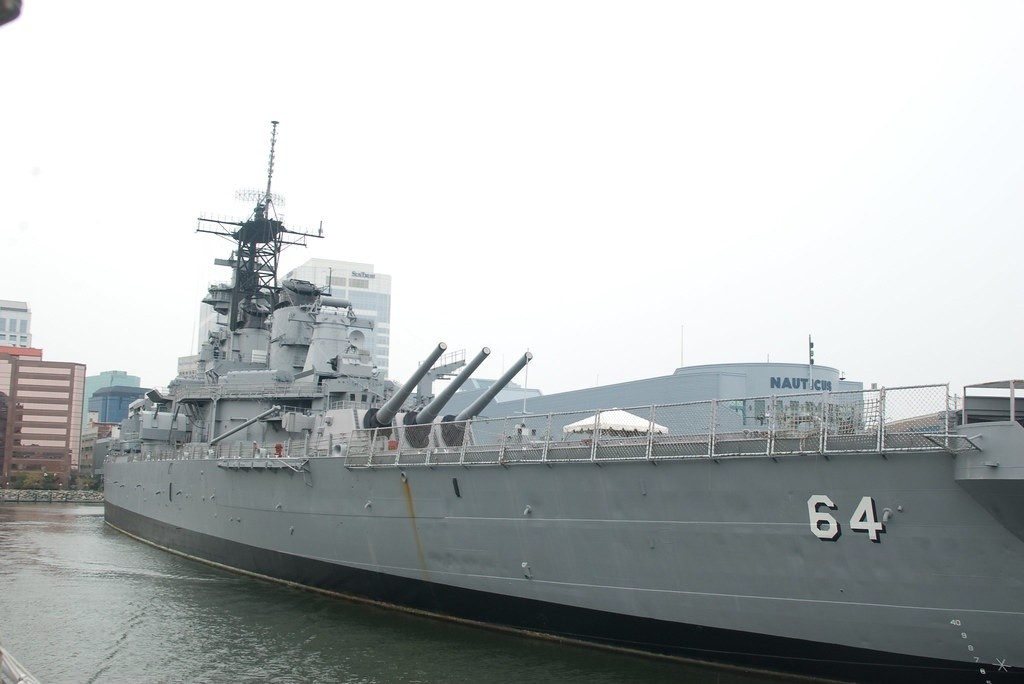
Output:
[{"left": 563, "top": 407, "right": 668, "bottom": 440}]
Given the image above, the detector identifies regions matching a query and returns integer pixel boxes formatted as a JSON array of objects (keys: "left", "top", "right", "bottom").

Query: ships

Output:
[{"left": 101, "top": 115, "right": 1023, "bottom": 684}]
